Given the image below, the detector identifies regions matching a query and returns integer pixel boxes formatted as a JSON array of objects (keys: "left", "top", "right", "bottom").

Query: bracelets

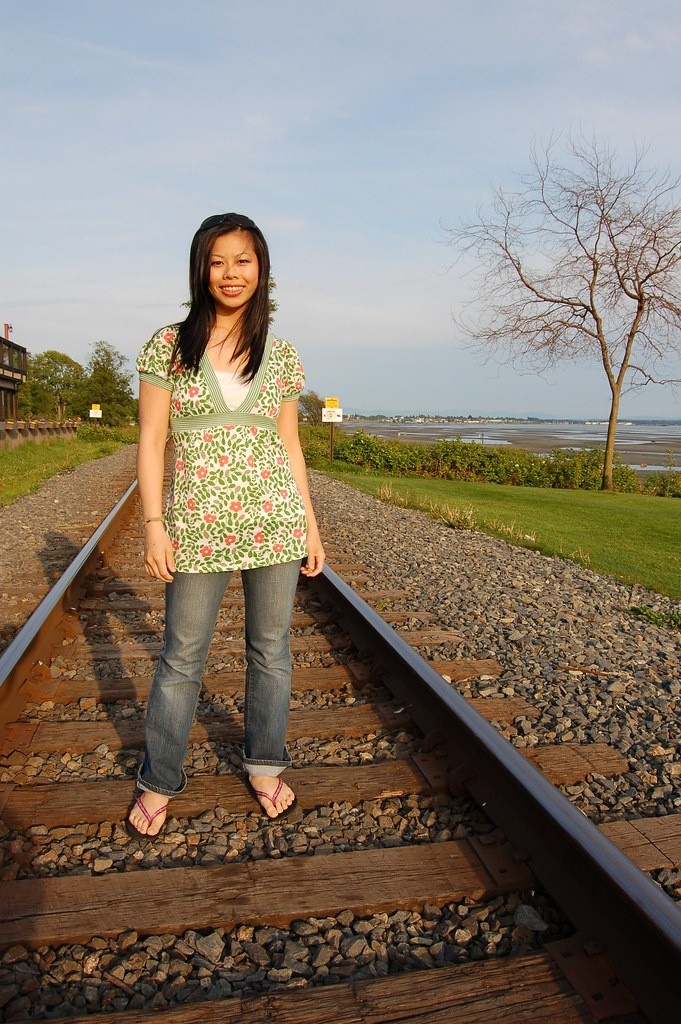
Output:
[{"left": 142, "top": 518, "right": 167, "bottom": 532}]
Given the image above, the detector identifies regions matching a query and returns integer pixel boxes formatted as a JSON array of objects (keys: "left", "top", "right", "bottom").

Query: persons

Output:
[{"left": 122, "top": 210, "right": 328, "bottom": 841}]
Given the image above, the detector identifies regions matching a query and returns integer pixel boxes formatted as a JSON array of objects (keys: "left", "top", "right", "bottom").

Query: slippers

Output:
[
  {"left": 255, "top": 779, "right": 296, "bottom": 819},
  {"left": 126, "top": 797, "right": 167, "bottom": 841}
]
